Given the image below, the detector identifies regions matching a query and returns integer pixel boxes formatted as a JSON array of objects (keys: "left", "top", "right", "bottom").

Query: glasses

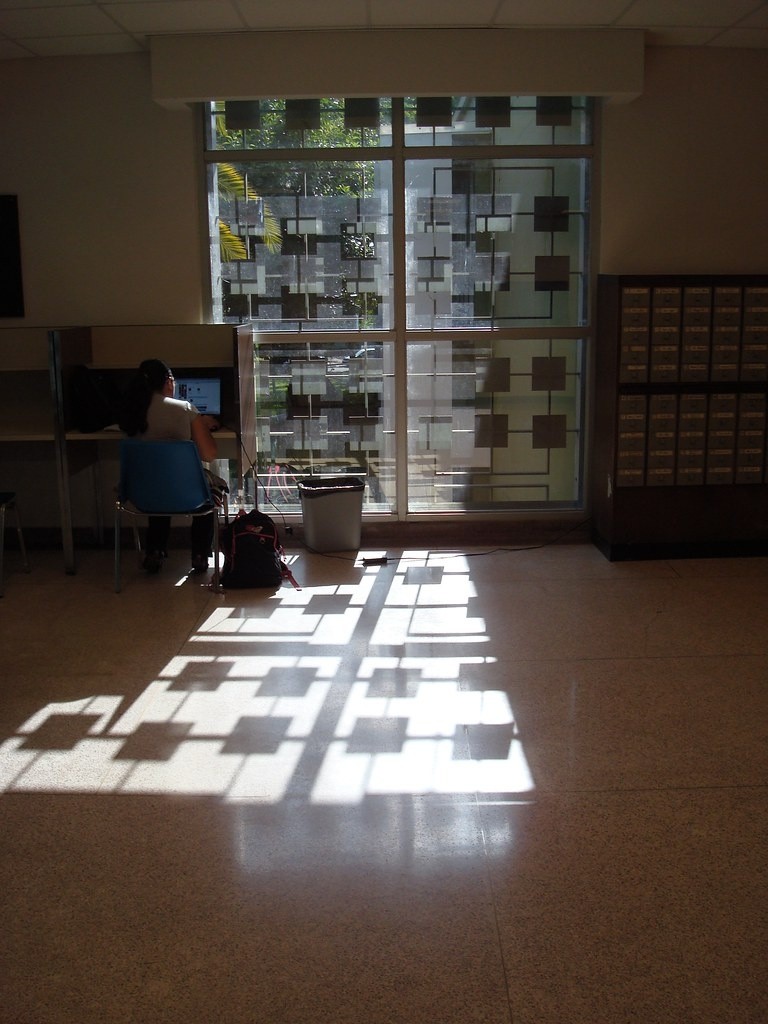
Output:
[{"left": 168, "top": 376, "right": 174, "bottom": 382}]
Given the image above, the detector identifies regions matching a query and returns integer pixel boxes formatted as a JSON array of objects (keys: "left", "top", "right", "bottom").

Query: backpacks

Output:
[{"left": 219, "top": 510, "right": 289, "bottom": 588}]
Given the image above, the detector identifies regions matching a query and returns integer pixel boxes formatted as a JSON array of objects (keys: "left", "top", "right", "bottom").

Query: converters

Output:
[{"left": 363, "top": 556, "right": 387, "bottom": 564}]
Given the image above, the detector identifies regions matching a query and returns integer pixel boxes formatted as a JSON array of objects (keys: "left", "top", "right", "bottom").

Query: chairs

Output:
[
  {"left": 111, "top": 439, "right": 228, "bottom": 594},
  {"left": 0, "top": 491, "right": 31, "bottom": 598}
]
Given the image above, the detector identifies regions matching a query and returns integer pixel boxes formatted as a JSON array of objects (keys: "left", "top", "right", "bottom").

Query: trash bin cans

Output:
[{"left": 296, "top": 475, "right": 367, "bottom": 555}]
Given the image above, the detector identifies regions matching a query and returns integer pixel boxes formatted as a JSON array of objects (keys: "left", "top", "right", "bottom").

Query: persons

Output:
[{"left": 118, "top": 359, "right": 229, "bottom": 575}]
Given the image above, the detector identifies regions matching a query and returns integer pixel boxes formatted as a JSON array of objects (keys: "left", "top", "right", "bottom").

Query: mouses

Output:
[{"left": 209, "top": 425, "right": 217, "bottom": 431}]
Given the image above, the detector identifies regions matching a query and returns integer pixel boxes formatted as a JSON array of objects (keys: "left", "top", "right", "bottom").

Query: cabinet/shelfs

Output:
[{"left": 594, "top": 272, "right": 768, "bottom": 543}]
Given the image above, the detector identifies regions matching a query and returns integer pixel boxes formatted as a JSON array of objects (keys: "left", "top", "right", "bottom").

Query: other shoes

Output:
[
  {"left": 191, "top": 551, "right": 208, "bottom": 570},
  {"left": 142, "top": 549, "right": 165, "bottom": 574}
]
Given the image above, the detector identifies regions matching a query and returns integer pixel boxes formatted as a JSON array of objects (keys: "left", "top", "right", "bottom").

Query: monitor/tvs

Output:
[{"left": 172, "top": 377, "right": 221, "bottom": 418}]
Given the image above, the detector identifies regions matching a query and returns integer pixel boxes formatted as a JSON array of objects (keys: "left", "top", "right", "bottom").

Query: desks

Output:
[{"left": 0, "top": 371, "right": 259, "bottom": 593}]
